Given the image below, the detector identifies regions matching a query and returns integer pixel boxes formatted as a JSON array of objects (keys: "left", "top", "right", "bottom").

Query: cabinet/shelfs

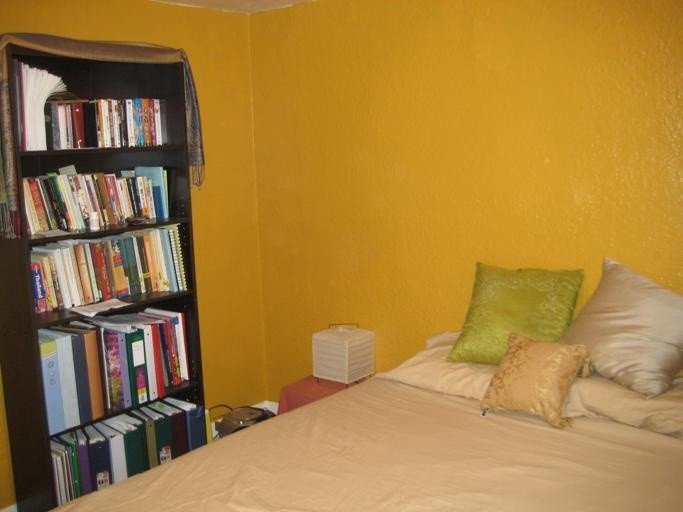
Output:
[{"left": 0, "top": 32, "right": 209, "bottom": 512}]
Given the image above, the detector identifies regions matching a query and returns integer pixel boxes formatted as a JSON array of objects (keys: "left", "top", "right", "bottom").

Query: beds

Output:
[{"left": 41, "top": 330, "right": 683, "bottom": 512}]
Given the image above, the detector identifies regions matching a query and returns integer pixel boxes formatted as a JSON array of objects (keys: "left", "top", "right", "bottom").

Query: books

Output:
[
  {"left": 8, "top": 56, "right": 173, "bottom": 152},
  {"left": 24, "top": 165, "right": 215, "bottom": 509}
]
